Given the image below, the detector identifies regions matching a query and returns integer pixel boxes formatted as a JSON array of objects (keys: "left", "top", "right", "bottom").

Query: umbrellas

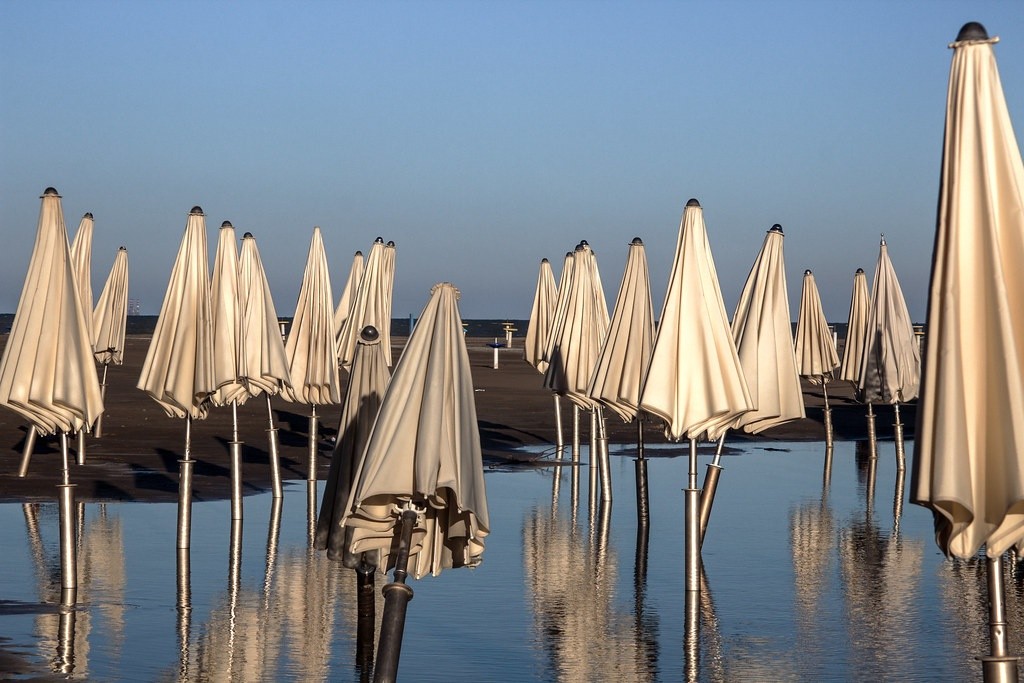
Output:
[
  {"left": 906, "top": 22, "right": 1024, "bottom": 683},
  {"left": 3, "top": 186, "right": 923, "bottom": 683}
]
